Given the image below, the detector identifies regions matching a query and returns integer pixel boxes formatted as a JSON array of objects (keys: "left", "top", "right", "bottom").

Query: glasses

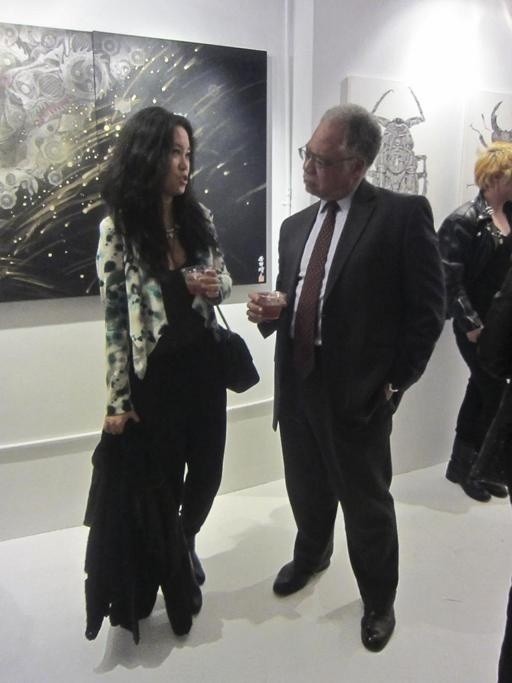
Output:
[{"left": 295, "top": 139, "right": 355, "bottom": 168}]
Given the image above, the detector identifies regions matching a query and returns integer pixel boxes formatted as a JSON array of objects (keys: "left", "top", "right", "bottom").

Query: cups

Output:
[
  {"left": 179, "top": 265, "right": 212, "bottom": 294},
  {"left": 256, "top": 288, "right": 288, "bottom": 321}
]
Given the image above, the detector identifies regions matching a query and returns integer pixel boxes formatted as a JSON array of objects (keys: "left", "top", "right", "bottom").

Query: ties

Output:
[{"left": 286, "top": 199, "right": 347, "bottom": 386}]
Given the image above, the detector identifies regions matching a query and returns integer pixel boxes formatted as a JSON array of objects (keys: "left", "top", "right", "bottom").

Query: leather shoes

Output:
[
  {"left": 357, "top": 600, "right": 398, "bottom": 653},
  {"left": 189, "top": 534, "right": 210, "bottom": 584},
  {"left": 184, "top": 577, "right": 202, "bottom": 615},
  {"left": 482, "top": 477, "right": 509, "bottom": 499},
  {"left": 271, "top": 555, "right": 333, "bottom": 600},
  {"left": 443, "top": 468, "right": 490, "bottom": 503}
]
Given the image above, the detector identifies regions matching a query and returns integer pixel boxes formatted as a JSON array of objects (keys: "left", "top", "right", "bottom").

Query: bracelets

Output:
[{"left": 387, "top": 381, "right": 400, "bottom": 392}]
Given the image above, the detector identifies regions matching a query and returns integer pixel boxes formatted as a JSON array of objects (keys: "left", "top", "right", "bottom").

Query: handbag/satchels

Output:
[{"left": 210, "top": 288, "right": 261, "bottom": 395}]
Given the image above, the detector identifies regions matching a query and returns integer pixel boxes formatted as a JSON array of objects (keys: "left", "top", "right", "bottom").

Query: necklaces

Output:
[{"left": 166, "top": 227, "right": 177, "bottom": 239}]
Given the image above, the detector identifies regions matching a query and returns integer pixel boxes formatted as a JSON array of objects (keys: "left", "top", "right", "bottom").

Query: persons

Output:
[
  {"left": 244, "top": 102, "right": 450, "bottom": 654},
  {"left": 81, "top": 107, "right": 261, "bottom": 645},
  {"left": 436, "top": 140, "right": 512, "bottom": 375}
]
